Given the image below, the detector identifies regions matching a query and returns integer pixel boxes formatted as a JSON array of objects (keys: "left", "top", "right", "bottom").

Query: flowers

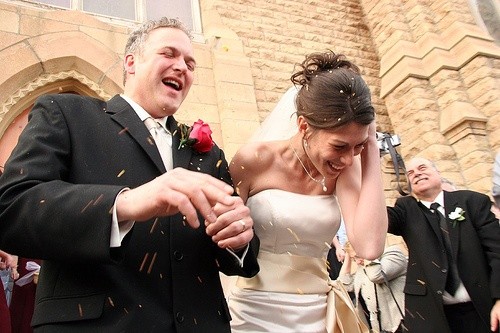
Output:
[
  {"left": 448, "top": 207, "right": 466, "bottom": 227},
  {"left": 177, "top": 119, "right": 215, "bottom": 154}
]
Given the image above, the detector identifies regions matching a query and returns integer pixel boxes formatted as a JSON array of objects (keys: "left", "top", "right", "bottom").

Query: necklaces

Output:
[{"left": 287, "top": 137, "right": 328, "bottom": 193}]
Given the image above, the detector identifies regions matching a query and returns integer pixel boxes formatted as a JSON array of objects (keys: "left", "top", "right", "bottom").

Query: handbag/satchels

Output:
[{"left": 380, "top": 320, "right": 408, "bottom": 333}]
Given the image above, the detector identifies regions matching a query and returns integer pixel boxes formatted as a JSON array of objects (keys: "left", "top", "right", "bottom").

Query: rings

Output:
[{"left": 238, "top": 219, "right": 249, "bottom": 231}]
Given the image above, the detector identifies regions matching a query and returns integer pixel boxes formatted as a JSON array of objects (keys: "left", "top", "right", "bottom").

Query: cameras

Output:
[{"left": 376, "top": 132, "right": 401, "bottom": 149}]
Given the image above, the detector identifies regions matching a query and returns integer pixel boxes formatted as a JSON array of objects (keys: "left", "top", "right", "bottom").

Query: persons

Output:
[
  {"left": 0, "top": 16, "right": 256, "bottom": 333},
  {"left": 489, "top": 200, "right": 500, "bottom": 221},
  {"left": 326, "top": 235, "right": 345, "bottom": 281},
  {"left": 18, "top": 257, "right": 44, "bottom": 333},
  {"left": 385, "top": 154, "right": 500, "bottom": 333},
  {"left": 440, "top": 177, "right": 457, "bottom": 193},
  {"left": 338, "top": 232, "right": 408, "bottom": 333},
  {"left": 228, "top": 50, "right": 389, "bottom": 333},
  {"left": 0, "top": 249, "right": 18, "bottom": 333}
]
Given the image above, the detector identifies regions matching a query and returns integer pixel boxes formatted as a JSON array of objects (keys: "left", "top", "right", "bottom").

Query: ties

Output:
[
  {"left": 144, "top": 117, "right": 173, "bottom": 172},
  {"left": 430, "top": 202, "right": 461, "bottom": 297}
]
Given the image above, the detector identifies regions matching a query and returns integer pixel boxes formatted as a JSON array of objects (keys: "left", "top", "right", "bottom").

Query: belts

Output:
[{"left": 444, "top": 305, "right": 474, "bottom": 314}]
